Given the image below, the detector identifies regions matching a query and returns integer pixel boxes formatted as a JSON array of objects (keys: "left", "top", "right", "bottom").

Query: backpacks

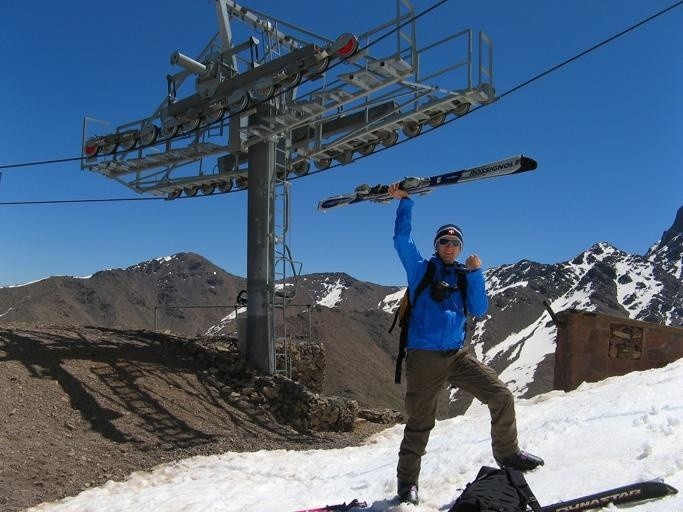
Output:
[
  {"left": 449, "top": 465, "right": 542, "bottom": 511},
  {"left": 398, "top": 262, "right": 469, "bottom": 324}
]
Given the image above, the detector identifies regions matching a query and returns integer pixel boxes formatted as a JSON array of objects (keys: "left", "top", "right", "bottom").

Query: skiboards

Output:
[
  {"left": 526, "top": 482, "right": 677, "bottom": 512},
  {"left": 317, "top": 156, "right": 536, "bottom": 212}
]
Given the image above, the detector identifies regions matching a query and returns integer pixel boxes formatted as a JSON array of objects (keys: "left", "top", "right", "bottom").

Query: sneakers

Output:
[
  {"left": 398, "top": 482, "right": 419, "bottom": 505},
  {"left": 501, "top": 450, "right": 544, "bottom": 474}
]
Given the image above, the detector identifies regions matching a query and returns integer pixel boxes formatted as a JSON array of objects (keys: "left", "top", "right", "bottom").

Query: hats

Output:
[{"left": 434, "top": 224, "right": 463, "bottom": 252}]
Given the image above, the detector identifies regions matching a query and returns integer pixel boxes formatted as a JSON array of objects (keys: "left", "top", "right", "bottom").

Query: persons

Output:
[{"left": 387, "top": 180, "right": 546, "bottom": 505}]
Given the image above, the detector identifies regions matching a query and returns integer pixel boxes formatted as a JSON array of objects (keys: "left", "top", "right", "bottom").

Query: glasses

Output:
[{"left": 439, "top": 237, "right": 460, "bottom": 247}]
